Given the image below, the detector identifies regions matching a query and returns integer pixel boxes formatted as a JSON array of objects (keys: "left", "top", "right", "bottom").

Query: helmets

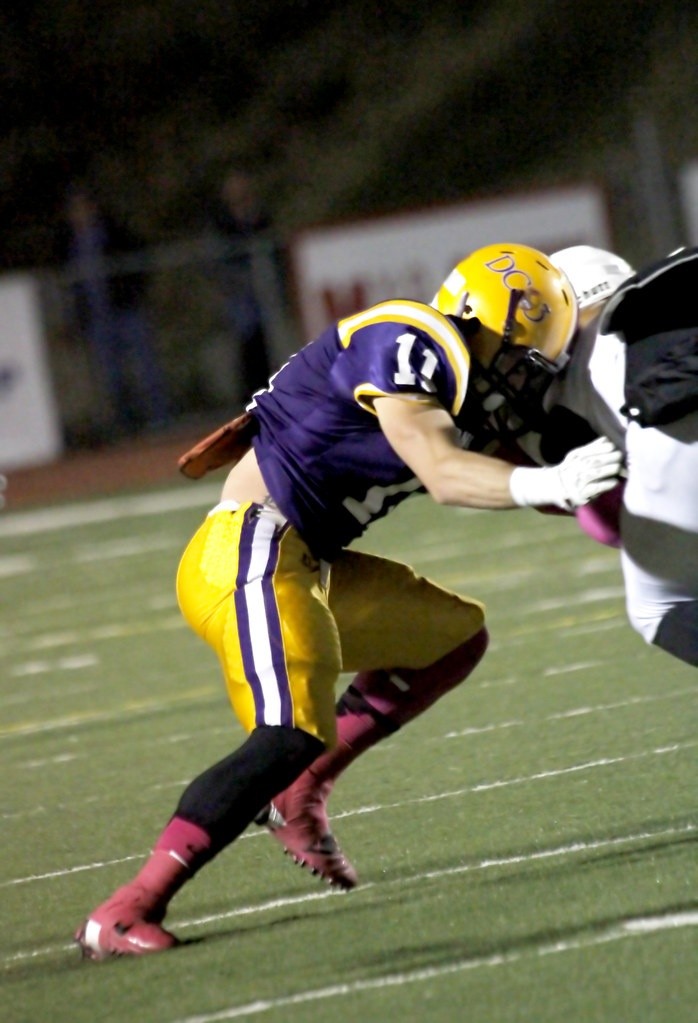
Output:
[{"left": 425, "top": 246, "right": 576, "bottom": 434}]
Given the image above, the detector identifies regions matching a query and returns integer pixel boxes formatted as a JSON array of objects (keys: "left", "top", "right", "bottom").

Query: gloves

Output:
[{"left": 508, "top": 433, "right": 625, "bottom": 507}]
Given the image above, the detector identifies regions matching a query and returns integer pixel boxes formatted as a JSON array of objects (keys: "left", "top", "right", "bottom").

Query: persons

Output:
[
  {"left": 65, "top": 191, "right": 169, "bottom": 430},
  {"left": 73, "top": 243, "right": 623, "bottom": 960},
  {"left": 464, "top": 246, "right": 698, "bottom": 666},
  {"left": 223, "top": 168, "right": 289, "bottom": 404}
]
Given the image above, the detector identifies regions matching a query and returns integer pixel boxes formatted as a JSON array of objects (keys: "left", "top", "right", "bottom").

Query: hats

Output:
[{"left": 548, "top": 245, "right": 633, "bottom": 307}]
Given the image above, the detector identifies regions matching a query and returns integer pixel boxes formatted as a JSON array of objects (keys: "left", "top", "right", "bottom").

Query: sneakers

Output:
[
  {"left": 258, "top": 774, "right": 359, "bottom": 892},
  {"left": 71, "top": 890, "right": 177, "bottom": 958}
]
